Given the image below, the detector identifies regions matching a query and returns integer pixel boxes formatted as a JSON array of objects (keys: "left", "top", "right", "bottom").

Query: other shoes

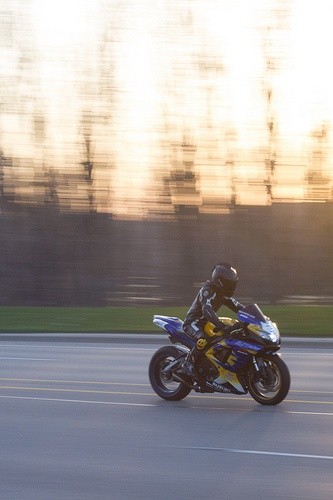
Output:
[{"left": 191, "top": 368, "right": 215, "bottom": 393}]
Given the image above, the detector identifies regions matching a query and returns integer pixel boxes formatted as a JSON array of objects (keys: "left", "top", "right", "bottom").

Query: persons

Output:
[{"left": 182, "top": 263, "right": 248, "bottom": 376}]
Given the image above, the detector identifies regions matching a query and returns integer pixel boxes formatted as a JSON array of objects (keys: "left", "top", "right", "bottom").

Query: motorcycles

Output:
[{"left": 149, "top": 303, "right": 291, "bottom": 405}]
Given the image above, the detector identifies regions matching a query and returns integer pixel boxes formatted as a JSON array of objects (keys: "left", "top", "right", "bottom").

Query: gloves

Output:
[{"left": 217, "top": 322, "right": 235, "bottom": 334}]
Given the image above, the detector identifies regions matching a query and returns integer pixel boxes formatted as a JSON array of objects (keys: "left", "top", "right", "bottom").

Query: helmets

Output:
[{"left": 212, "top": 263, "right": 238, "bottom": 297}]
